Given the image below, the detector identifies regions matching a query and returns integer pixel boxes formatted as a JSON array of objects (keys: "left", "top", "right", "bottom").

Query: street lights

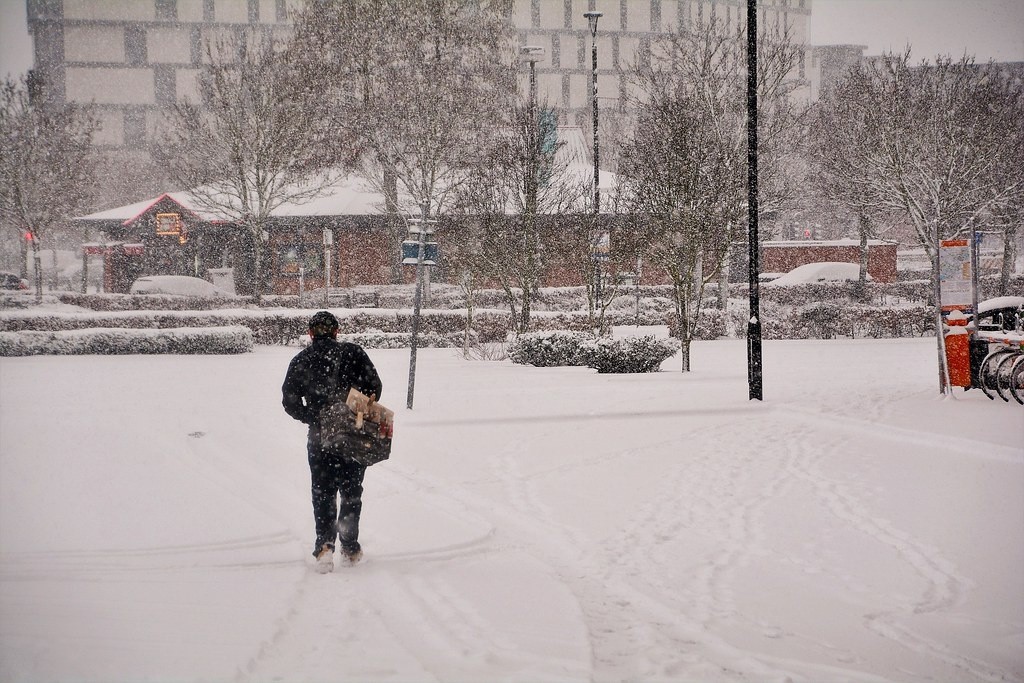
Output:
[
  {"left": 583, "top": 13, "right": 604, "bottom": 312},
  {"left": 521, "top": 46, "right": 545, "bottom": 300}
]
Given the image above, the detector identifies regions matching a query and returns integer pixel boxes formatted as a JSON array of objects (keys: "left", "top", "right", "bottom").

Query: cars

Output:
[{"left": 940, "top": 296, "right": 1024, "bottom": 389}]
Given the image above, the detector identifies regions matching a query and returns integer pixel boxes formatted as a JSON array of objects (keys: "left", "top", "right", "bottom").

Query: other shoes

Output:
[
  {"left": 341, "top": 549, "right": 362, "bottom": 567},
  {"left": 316, "top": 541, "right": 335, "bottom": 575}
]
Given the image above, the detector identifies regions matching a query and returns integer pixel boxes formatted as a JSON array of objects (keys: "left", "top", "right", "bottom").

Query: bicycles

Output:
[
  {"left": 979, "top": 348, "right": 1024, "bottom": 400},
  {"left": 1010, "top": 355, "right": 1024, "bottom": 404},
  {"left": 995, "top": 353, "right": 1021, "bottom": 403}
]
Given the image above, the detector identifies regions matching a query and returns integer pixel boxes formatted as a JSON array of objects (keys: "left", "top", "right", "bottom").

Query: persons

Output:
[{"left": 282, "top": 311, "right": 384, "bottom": 578}]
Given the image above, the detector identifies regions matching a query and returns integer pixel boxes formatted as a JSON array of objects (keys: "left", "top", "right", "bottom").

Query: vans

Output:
[{"left": 130, "top": 276, "right": 238, "bottom": 298}]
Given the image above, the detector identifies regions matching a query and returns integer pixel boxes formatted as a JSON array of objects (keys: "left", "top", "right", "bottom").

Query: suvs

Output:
[{"left": 1, "top": 272, "right": 28, "bottom": 290}]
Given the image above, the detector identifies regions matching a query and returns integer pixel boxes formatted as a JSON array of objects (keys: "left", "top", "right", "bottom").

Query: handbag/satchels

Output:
[{"left": 319, "top": 386, "right": 393, "bottom": 466}]
